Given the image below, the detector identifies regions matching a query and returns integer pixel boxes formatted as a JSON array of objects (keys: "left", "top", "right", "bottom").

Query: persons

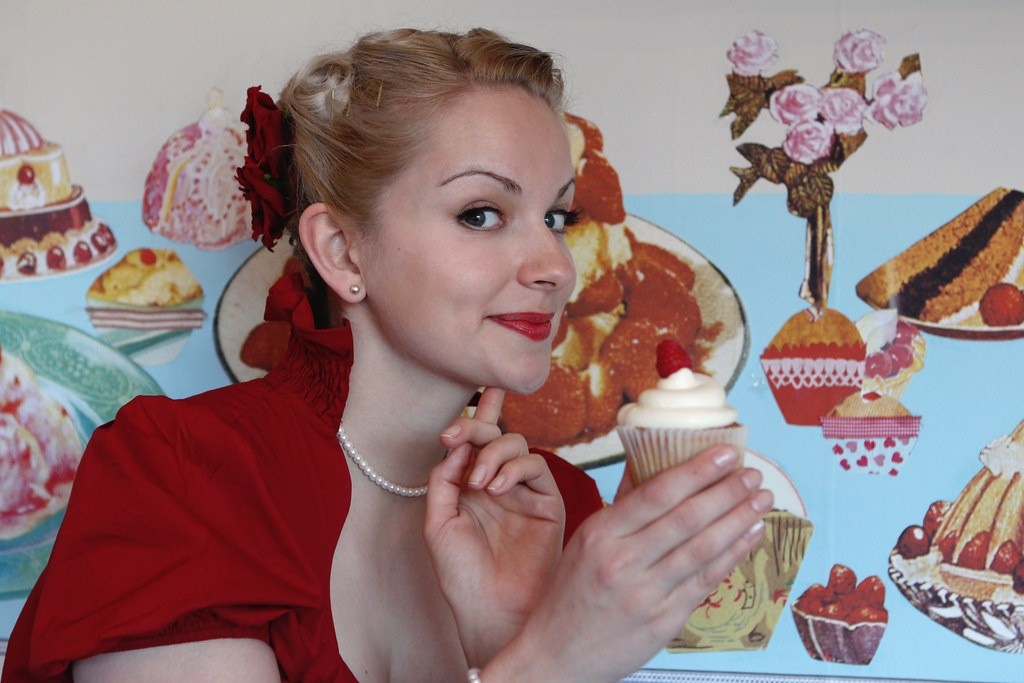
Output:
[{"left": 0, "top": 28, "right": 773, "bottom": 683}]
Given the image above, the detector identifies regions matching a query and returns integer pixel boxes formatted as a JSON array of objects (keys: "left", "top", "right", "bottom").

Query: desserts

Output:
[{"left": 612, "top": 340, "right": 746, "bottom": 488}]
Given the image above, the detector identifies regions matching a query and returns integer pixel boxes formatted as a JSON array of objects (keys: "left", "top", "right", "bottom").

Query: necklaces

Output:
[{"left": 334, "top": 425, "right": 433, "bottom": 497}]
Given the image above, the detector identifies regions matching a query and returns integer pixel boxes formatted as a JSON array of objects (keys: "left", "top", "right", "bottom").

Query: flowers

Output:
[{"left": 720, "top": 28, "right": 927, "bottom": 309}]
